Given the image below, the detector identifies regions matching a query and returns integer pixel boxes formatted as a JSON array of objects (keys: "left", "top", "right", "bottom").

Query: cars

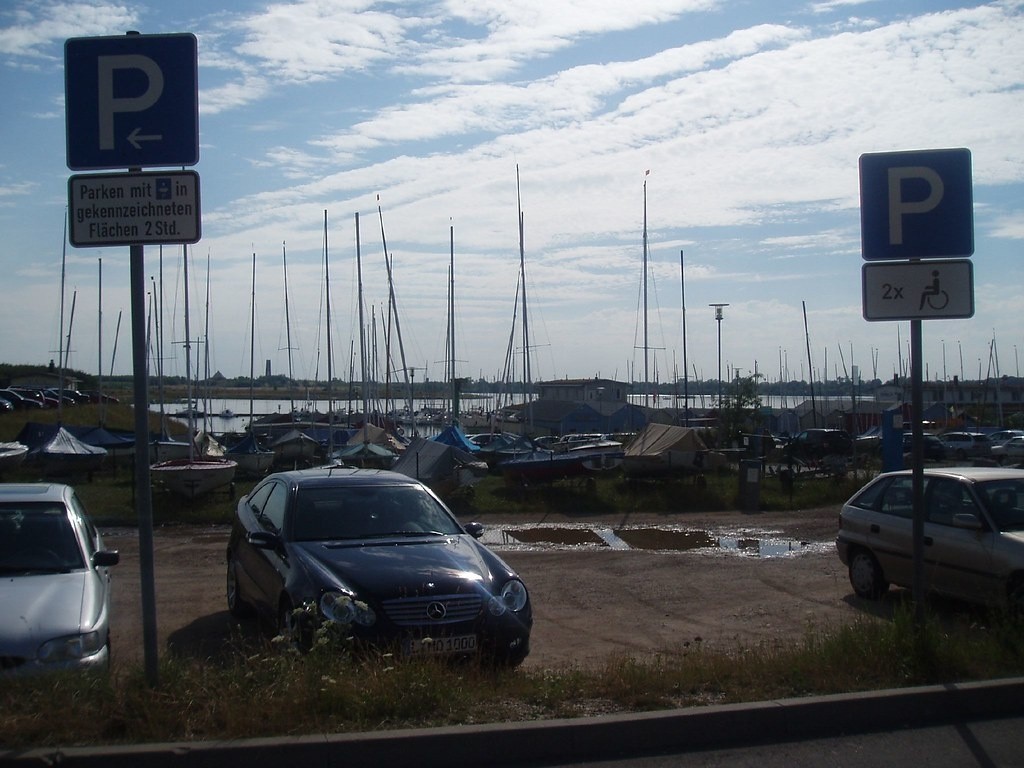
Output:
[
  {"left": 835, "top": 466, "right": 1023, "bottom": 615},
  {"left": 227, "top": 466, "right": 532, "bottom": 672},
  {"left": 0, "top": 482, "right": 120, "bottom": 689}
]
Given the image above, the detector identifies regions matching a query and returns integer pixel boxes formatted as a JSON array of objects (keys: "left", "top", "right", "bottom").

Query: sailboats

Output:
[{"left": 1, "top": 160, "right": 1022, "bottom": 500}]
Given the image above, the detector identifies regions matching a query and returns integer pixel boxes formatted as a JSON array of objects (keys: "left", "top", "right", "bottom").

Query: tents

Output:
[
  {"left": 430, "top": 424, "right": 625, "bottom": 481},
  {"left": 19, "top": 420, "right": 175, "bottom": 478},
  {"left": 391, "top": 437, "right": 489, "bottom": 484},
  {"left": 225, "top": 421, "right": 409, "bottom": 471},
  {"left": 622, "top": 422, "right": 708, "bottom": 474}
]
